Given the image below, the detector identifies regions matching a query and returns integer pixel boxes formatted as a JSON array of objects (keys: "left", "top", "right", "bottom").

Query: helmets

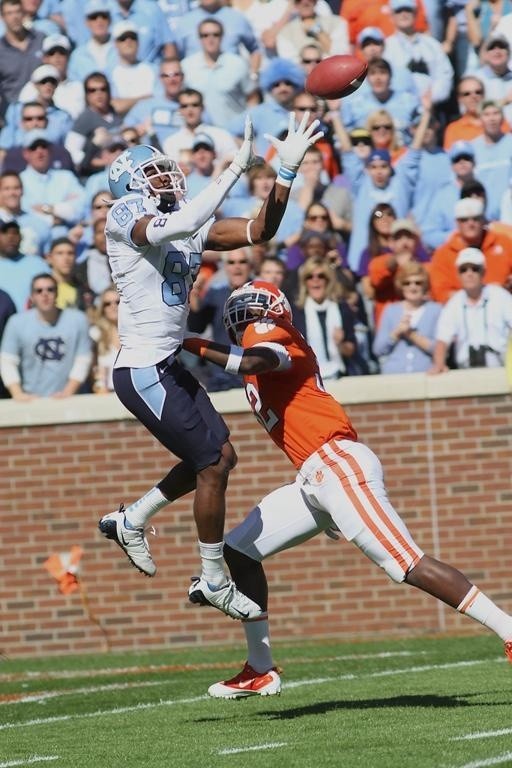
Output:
[
  {"left": 223, "top": 280, "right": 293, "bottom": 346},
  {"left": 109, "top": 144, "right": 187, "bottom": 207}
]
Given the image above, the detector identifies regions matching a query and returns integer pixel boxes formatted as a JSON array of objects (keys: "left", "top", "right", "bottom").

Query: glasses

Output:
[
  {"left": 228, "top": 260, "right": 246, "bottom": 264},
  {"left": 160, "top": 33, "right": 220, "bottom": 108},
  {"left": 303, "top": 61, "right": 320, "bottom": 64},
  {"left": 309, "top": 216, "right": 328, "bottom": 222},
  {"left": 306, "top": 274, "right": 325, "bottom": 279},
  {"left": 402, "top": 282, "right": 421, "bottom": 285},
  {"left": 104, "top": 301, "right": 120, "bottom": 306},
  {"left": 459, "top": 268, "right": 479, "bottom": 273},
  {"left": 351, "top": 137, "right": 371, "bottom": 146},
  {"left": 37, "top": 288, "right": 54, "bottom": 294},
  {"left": 372, "top": 125, "right": 391, "bottom": 130},
  {"left": 464, "top": 91, "right": 481, "bottom": 96}
]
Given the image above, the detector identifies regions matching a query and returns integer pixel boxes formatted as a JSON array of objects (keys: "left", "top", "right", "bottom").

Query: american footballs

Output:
[{"left": 306, "top": 56, "right": 368, "bottom": 99}]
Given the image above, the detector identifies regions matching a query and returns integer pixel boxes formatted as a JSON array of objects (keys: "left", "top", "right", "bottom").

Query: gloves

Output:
[
  {"left": 263, "top": 110, "right": 325, "bottom": 188},
  {"left": 229, "top": 115, "right": 265, "bottom": 177}
]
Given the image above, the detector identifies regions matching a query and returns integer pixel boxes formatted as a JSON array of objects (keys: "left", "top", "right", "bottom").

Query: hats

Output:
[
  {"left": 21, "top": 3, "right": 138, "bottom": 149},
  {"left": 358, "top": 26, "right": 384, "bottom": 46},
  {"left": 190, "top": 135, "right": 215, "bottom": 151},
  {"left": 486, "top": 31, "right": 509, "bottom": 50},
  {"left": 258, "top": 57, "right": 306, "bottom": 93},
  {"left": 389, "top": 0, "right": 417, "bottom": 11},
  {"left": 366, "top": 140, "right": 485, "bottom": 268}
]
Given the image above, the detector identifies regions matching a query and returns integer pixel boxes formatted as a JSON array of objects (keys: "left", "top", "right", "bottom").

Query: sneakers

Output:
[
  {"left": 207, "top": 661, "right": 284, "bottom": 699},
  {"left": 98, "top": 503, "right": 157, "bottom": 577},
  {"left": 188, "top": 576, "right": 262, "bottom": 620}
]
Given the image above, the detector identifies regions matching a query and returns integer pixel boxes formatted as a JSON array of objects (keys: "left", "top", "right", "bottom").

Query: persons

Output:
[
  {"left": 179, "top": 282, "right": 512, "bottom": 698},
  {"left": 99, "top": 106, "right": 326, "bottom": 622}
]
